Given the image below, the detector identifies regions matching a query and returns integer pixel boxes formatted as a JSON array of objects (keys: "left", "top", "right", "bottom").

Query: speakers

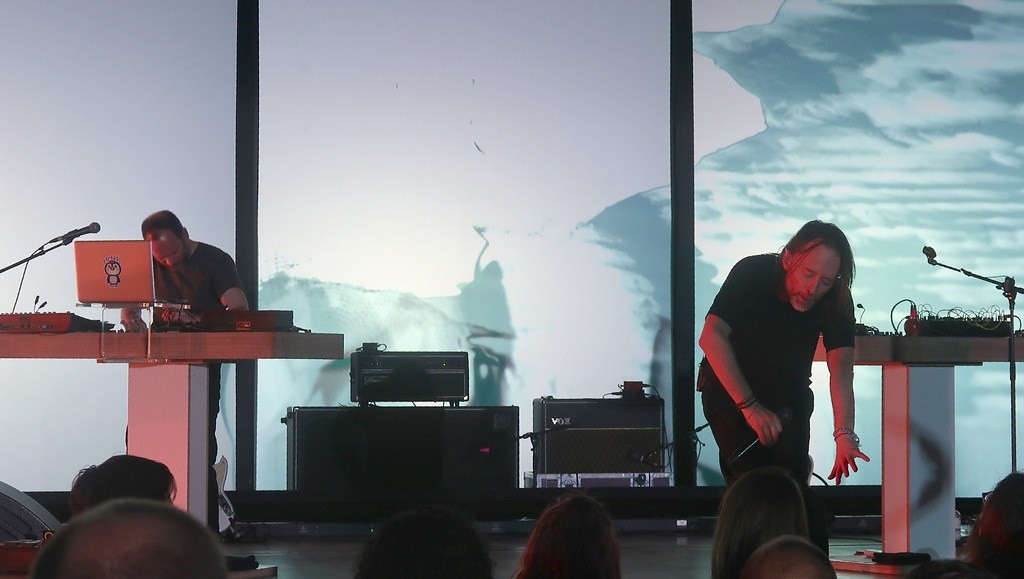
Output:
[
  {"left": 532, "top": 398, "right": 664, "bottom": 473},
  {"left": 286, "top": 406, "right": 519, "bottom": 512},
  {"left": 0, "top": 481, "right": 61, "bottom": 541},
  {"left": 350, "top": 351, "right": 469, "bottom": 403}
]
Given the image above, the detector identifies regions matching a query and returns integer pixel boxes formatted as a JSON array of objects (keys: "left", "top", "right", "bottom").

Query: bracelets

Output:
[
  {"left": 737, "top": 393, "right": 759, "bottom": 410},
  {"left": 833, "top": 427, "right": 860, "bottom": 445}
]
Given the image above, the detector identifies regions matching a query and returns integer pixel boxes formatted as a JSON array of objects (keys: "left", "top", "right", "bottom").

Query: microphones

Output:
[
  {"left": 48, "top": 222, "right": 100, "bottom": 244},
  {"left": 729, "top": 407, "right": 793, "bottom": 464}
]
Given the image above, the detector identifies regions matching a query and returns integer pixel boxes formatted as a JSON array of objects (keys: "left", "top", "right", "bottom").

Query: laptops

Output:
[{"left": 73, "top": 241, "right": 188, "bottom": 305}]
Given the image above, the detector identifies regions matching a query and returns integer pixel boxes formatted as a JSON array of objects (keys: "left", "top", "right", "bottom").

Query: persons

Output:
[
  {"left": 27, "top": 455, "right": 1023, "bottom": 579},
  {"left": 121, "top": 211, "right": 250, "bottom": 543},
  {"left": 698, "top": 219, "right": 870, "bottom": 486}
]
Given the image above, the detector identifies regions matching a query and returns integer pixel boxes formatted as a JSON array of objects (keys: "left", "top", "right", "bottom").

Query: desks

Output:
[
  {"left": 0, "top": 332, "right": 344, "bottom": 529},
  {"left": 75, "top": 302, "right": 192, "bottom": 362},
  {"left": 812, "top": 336, "right": 1024, "bottom": 561}
]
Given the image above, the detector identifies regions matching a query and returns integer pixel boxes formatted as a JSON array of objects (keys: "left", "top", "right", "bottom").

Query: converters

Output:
[
  {"left": 363, "top": 342, "right": 378, "bottom": 351},
  {"left": 620, "top": 381, "right": 645, "bottom": 398}
]
